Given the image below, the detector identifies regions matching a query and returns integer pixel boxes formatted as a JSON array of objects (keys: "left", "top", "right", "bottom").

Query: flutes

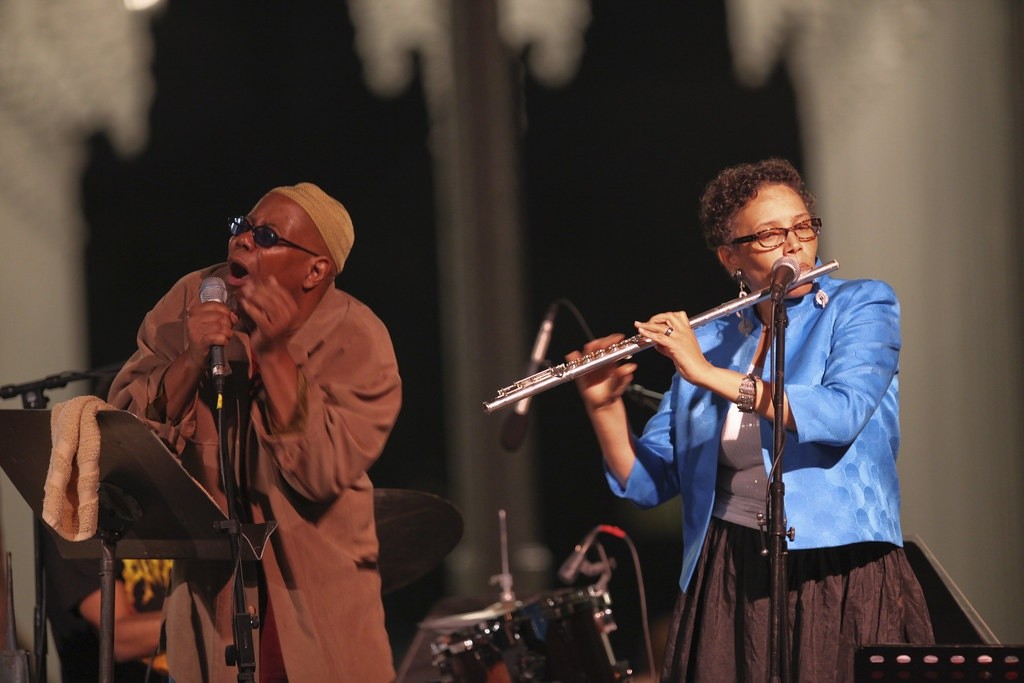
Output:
[{"left": 484, "top": 259, "right": 840, "bottom": 414}]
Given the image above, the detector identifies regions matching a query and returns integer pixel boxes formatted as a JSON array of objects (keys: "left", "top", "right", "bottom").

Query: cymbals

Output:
[{"left": 373, "top": 488, "right": 466, "bottom": 592}]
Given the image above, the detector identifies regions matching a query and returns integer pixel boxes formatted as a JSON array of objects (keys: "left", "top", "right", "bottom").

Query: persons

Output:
[
  {"left": 105, "top": 182, "right": 403, "bottom": 682},
  {"left": 35, "top": 532, "right": 168, "bottom": 683},
  {"left": 564, "top": 158, "right": 937, "bottom": 683}
]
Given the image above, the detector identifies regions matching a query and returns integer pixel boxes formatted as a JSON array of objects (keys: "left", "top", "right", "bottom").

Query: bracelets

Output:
[{"left": 735, "top": 373, "right": 759, "bottom": 413}]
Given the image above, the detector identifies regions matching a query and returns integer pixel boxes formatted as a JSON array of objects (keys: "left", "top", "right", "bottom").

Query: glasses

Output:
[
  {"left": 224, "top": 216, "right": 319, "bottom": 257},
  {"left": 710, "top": 218, "right": 823, "bottom": 248}
]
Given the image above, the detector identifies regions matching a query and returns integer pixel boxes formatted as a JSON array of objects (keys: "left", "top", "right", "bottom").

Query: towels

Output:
[{"left": 39, "top": 392, "right": 147, "bottom": 543}]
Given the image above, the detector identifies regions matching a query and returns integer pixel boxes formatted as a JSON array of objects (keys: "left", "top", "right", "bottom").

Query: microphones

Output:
[
  {"left": 198, "top": 277, "right": 228, "bottom": 393},
  {"left": 501, "top": 307, "right": 557, "bottom": 454},
  {"left": 559, "top": 532, "right": 601, "bottom": 586},
  {"left": 770, "top": 257, "right": 800, "bottom": 302}
]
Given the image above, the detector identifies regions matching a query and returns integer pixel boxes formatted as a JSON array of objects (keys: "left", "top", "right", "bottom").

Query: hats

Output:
[{"left": 269, "top": 182, "right": 355, "bottom": 275}]
